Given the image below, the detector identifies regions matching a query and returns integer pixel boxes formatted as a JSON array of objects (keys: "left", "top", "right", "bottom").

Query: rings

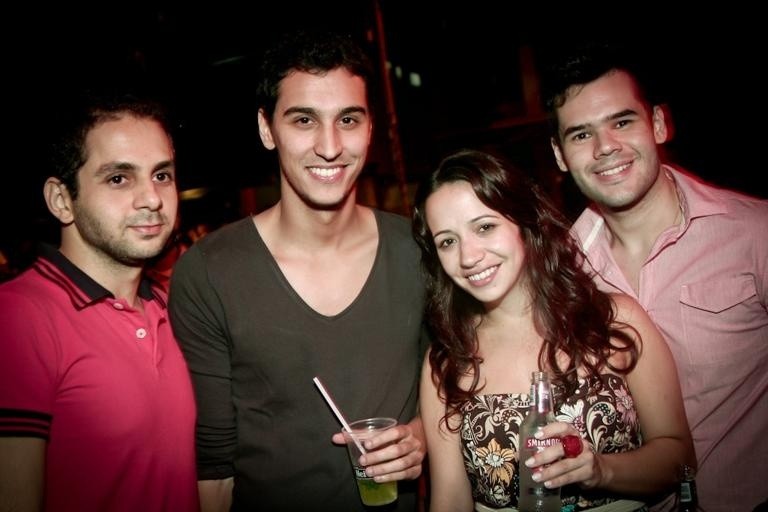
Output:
[{"left": 562, "top": 435, "right": 583, "bottom": 457}]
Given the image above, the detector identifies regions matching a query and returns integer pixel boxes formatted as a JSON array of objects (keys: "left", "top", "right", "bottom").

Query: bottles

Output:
[
  {"left": 518, "top": 370, "right": 568, "bottom": 512},
  {"left": 671, "top": 461, "right": 700, "bottom": 511}
]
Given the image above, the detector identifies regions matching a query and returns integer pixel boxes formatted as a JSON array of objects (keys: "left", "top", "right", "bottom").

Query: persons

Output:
[
  {"left": 412, "top": 151, "right": 696, "bottom": 512},
  {"left": 547, "top": 48, "right": 768, "bottom": 512},
  {"left": 168, "top": 33, "right": 427, "bottom": 512},
  {"left": 0, "top": 84, "right": 201, "bottom": 512}
]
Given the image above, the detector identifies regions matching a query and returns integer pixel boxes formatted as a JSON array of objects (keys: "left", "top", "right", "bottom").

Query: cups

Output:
[{"left": 339, "top": 415, "right": 403, "bottom": 507}]
